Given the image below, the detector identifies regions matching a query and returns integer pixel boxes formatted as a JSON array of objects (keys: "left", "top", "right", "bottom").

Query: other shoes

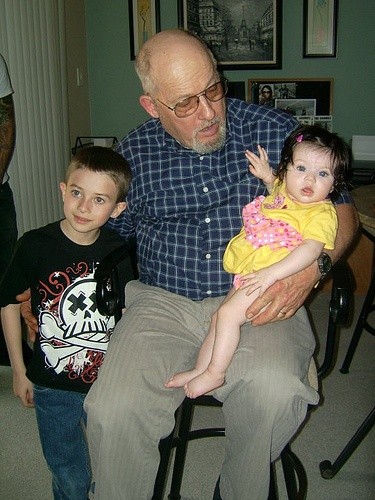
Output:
[{"left": 0, "top": 341, "right": 32, "bottom": 366}]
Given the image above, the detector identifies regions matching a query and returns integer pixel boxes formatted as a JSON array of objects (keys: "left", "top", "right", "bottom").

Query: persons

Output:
[
  {"left": 163, "top": 123, "right": 352, "bottom": 399},
  {"left": 258, "top": 85, "right": 275, "bottom": 109},
  {"left": 84, "top": 27, "right": 361, "bottom": 500},
  {"left": 0, "top": 146, "right": 140, "bottom": 500},
  {"left": 0, "top": 53, "right": 19, "bottom": 368}
]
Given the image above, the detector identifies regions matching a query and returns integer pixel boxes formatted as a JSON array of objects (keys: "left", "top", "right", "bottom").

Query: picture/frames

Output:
[
  {"left": 248, "top": 78, "right": 334, "bottom": 118},
  {"left": 302, "top": 0, "right": 338, "bottom": 58},
  {"left": 177, "top": 0, "right": 282, "bottom": 70},
  {"left": 127, "top": 0, "right": 161, "bottom": 61}
]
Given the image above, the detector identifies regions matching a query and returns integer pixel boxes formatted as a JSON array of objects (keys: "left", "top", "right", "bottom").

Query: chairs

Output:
[{"left": 153, "top": 260, "right": 353, "bottom": 500}]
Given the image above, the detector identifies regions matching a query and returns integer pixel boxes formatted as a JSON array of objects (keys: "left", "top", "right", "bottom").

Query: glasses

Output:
[{"left": 154, "top": 73, "right": 229, "bottom": 118}]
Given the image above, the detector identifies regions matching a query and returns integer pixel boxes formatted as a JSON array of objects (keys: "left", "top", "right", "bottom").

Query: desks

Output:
[{"left": 339, "top": 184, "right": 374, "bottom": 375}]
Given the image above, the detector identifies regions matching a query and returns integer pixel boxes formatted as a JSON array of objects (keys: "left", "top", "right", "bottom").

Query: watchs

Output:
[{"left": 316, "top": 251, "right": 333, "bottom": 282}]
